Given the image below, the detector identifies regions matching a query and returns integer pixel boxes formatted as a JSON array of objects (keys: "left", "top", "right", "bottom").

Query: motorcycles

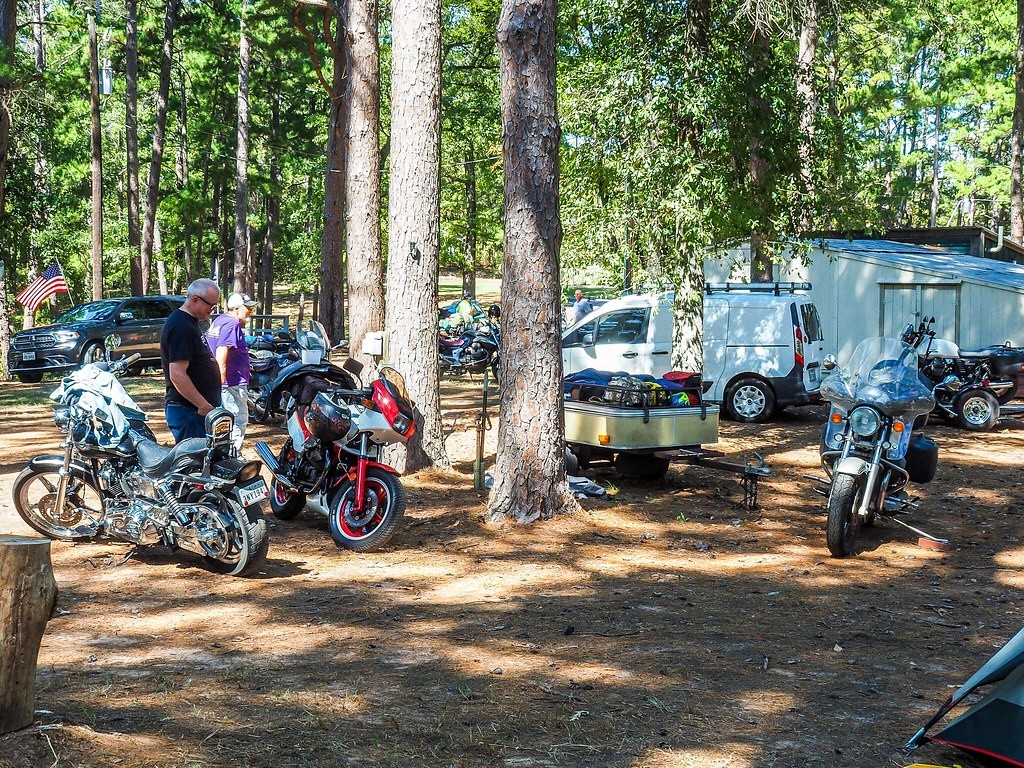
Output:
[
  {"left": 819, "top": 337, "right": 960, "bottom": 557},
  {"left": 12, "top": 333, "right": 270, "bottom": 577},
  {"left": 247, "top": 320, "right": 358, "bottom": 423},
  {"left": 868, "top": 316, "right": 1024, "bottom": 431},
  {"left": 439, "top": 305, "right": 500, "bottom": 380},
  {"left": 253, "top": 357, "right": 415, "bottom": 553}
]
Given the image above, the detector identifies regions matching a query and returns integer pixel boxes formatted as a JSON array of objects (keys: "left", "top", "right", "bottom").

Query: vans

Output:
[{"left": 562, "top": 282, "right": 824, "bottom": 424}]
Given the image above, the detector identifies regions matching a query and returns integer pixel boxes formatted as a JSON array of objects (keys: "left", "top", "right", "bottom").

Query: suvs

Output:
[{"left": 7, "top": 295, "right": 210, "bottom": 383}]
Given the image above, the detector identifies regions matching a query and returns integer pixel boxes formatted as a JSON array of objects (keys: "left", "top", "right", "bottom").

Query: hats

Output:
[{"left": 228, "top": 293, "right": 258, "bottom": 309}]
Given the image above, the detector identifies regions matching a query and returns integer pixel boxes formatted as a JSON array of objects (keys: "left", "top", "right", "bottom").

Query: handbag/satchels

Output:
[{"left": 564, "top": 368, "right": 703, "bottom": 410}]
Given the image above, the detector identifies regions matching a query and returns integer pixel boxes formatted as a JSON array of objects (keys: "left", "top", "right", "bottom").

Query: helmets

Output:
[
  {"left": 488, "top": 304, "right": 500, "bottom": 318},
  {"left": 304, "top": 391, "right": 351, "bottom": 441},
  {"left": 449, "top": 313, "right": 464, "bottom": 328},
  {"left": 464, "top": 293, "right": 471, "bottom": 298}
]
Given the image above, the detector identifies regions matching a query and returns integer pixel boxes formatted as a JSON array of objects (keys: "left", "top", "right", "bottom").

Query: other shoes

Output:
[{"left": 237, "top": 456, "right": 246, "bottom": 462}]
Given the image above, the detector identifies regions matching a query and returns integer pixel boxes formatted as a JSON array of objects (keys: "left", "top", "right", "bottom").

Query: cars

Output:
[
  {"left": 437, "top": 300, "right": 484, "bottom": 320},
  {"left": 559, "top": 298, "right": 647, "bottom": 339}
]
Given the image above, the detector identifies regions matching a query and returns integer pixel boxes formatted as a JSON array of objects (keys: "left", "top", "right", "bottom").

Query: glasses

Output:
[{"left": 194, "top": 295, "right": 218, "bottom": 310}]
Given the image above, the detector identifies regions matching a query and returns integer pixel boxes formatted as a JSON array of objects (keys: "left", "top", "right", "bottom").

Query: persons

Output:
[
  {"left": 574, "top": 290, "right": 592, "bottom": 323},
  {"left": 456, "top": 293, "right": 474, "bottom": 325},
  {"left": 206, "top": 293, "right": 258, "bottom": 457},
  {"left": 160, "top": 278, "right": 222, "bottom": 445}
]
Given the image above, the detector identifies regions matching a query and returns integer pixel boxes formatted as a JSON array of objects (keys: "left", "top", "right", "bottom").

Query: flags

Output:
[{"left": 16, "top": 259, "right": 67, "bottom": 311}]
